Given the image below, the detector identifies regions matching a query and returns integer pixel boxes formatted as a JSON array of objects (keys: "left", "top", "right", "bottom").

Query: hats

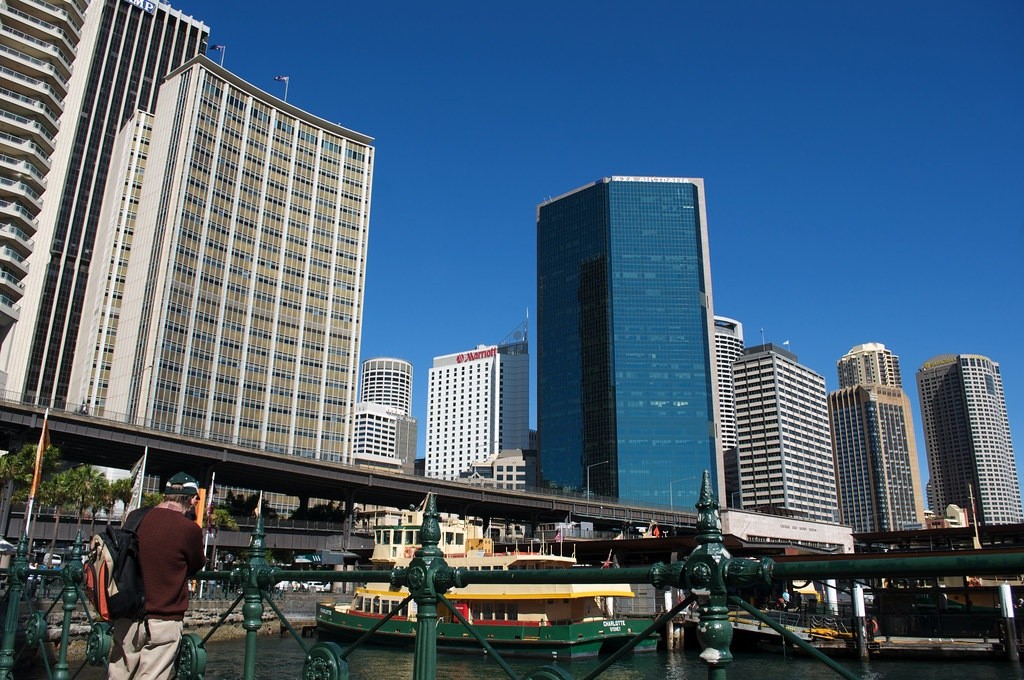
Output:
[{"left": 164, "top": 471, "right": 201, "bottom": 500}]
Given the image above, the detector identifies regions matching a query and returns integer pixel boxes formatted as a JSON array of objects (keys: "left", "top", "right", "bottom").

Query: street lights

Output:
[
  {"left": 328, "top": 411, "right": 354, "bottom": 461},
  {"left": 225, "top": 553, "right": 233, "bottom": 602},
  {"left": 587, "top": 461, "right": 608, "bottom": 502},
  {"left": 125, "top": 365, "right": 153, "bottom": 421},
  {"left": 473, "top": 440, "right": 498, "bottom": 477},
  {"left": 669, "top": 476, "right": 696, "bottom": 511},
  {"left": 731, "top": 488, "right": 756, "bottom": 509}
]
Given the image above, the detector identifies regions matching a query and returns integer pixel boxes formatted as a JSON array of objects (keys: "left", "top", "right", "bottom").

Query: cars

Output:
[
  {"left": 307, "top": 581, "right": 326, "bottom": 592},
  {"left": 323, "top": 582, "right": 333, "bottom": 592}
]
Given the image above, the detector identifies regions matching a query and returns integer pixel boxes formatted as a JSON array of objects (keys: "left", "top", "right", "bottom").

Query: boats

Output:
[
  {"left": 867, "top": 584, "right": 1024, "bottom": 639},
  {"left": 314, "top": 503, "right": 661, "bottom": 660},
  {"left": 683, "top": 590, "right": 880, "bottom": 655}
]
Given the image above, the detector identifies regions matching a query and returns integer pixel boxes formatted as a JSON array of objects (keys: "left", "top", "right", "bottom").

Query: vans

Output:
[{"left": 43, "top": 552, "right": 62, "bottom": 567}]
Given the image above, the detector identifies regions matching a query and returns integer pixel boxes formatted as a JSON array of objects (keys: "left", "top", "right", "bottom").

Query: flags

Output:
[
  {"left": 120, "top": 455, "right": 147, "bottom": 530},
  {"left": 24, "top": 420, "right": 51, "bottom": 538},
  {"left": 202, "top": 474, "right": 220, "bottom": 572},
  {"left": 555, "top": 532, "right": 561, "bottom": 542}
]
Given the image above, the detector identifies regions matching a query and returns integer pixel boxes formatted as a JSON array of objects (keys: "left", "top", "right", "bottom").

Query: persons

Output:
[
  {"left": 782, "top": 589, "right": 790, "bottom": 612},
  {"left": 108, "top": 472, "right": 207, "bottom": 680}
]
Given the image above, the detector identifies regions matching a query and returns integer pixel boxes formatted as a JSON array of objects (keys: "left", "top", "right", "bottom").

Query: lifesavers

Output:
[
  {"left": 865, "top": 620, "right": 878, "bottom": 633},
  {"left": 969, "top": 577, "right": 980, "bottom": 588}
]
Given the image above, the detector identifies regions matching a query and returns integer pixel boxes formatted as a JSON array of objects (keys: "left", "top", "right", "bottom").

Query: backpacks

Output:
[{"left": 83, "top": 505, "right": 154, "bottom": 639}]
